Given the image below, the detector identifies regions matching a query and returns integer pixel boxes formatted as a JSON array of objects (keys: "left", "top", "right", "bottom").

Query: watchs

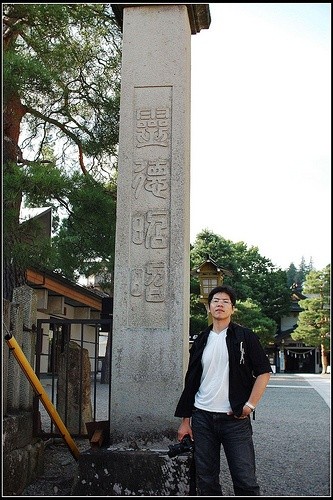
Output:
[{"left": 245, "top": 402, "right": 257, "bottom": 412}]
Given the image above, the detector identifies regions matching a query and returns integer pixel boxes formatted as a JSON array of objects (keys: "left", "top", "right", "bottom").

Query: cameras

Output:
[{"left": 168, "top": 434, "right": 195, "bottom": 458}]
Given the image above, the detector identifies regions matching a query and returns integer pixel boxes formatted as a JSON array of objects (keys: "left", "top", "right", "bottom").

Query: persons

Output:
[{"left": 173, "top": 283, "right": 274, "bottom": 495}]
